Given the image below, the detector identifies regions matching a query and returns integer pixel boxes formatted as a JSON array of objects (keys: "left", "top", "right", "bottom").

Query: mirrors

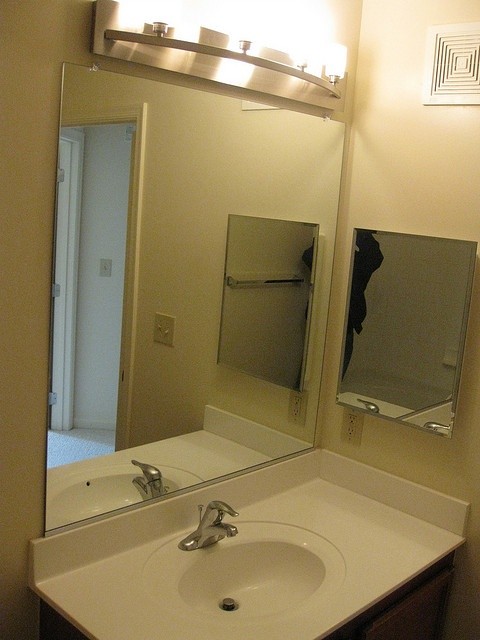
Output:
[
  {"left": 336, "top": 227, "right": 478, "bottom": 439},
  {"left": 43, "top": 62, "right": 346, "bottom": 531},
  {"left": 217, "top": 213, "right": 320, "bottom": 393}
]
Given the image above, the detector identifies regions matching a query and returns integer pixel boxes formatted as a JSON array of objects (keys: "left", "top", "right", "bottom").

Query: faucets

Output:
[
  {"left": 178, "top": 500, "right": 238, "bottom": 551},
  {"left": 357, "top": 397, "right": 380, "bottom": 414},
  {"left": 424, "top": 422, "right": 450, "bottom": 433},
  {"left": 130, "top": 459, "right": 169, "bottom": 500}
]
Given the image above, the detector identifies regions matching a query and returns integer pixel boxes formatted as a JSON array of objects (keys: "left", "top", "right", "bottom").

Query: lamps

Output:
[{"left": 324, "top": 43, "right": 348, "bottom": 87}]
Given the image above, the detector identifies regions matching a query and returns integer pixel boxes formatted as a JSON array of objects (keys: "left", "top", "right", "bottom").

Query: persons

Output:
[{"left": 341, "top": 230, "right": 383, "bottom": 382}]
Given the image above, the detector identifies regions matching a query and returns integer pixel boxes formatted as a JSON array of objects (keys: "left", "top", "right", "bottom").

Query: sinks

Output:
[
  {"left": 337, "top": 393, "right": 414, "bottom": 419},
  {"left": 403, "top": 402, "right": 451, "bottom": 437},
  {"left": 33, "top": 476, "right": 464, "bottom": 640},
  {"left": 46, "top": 429, "right": 272, "bottom": 528}
]
